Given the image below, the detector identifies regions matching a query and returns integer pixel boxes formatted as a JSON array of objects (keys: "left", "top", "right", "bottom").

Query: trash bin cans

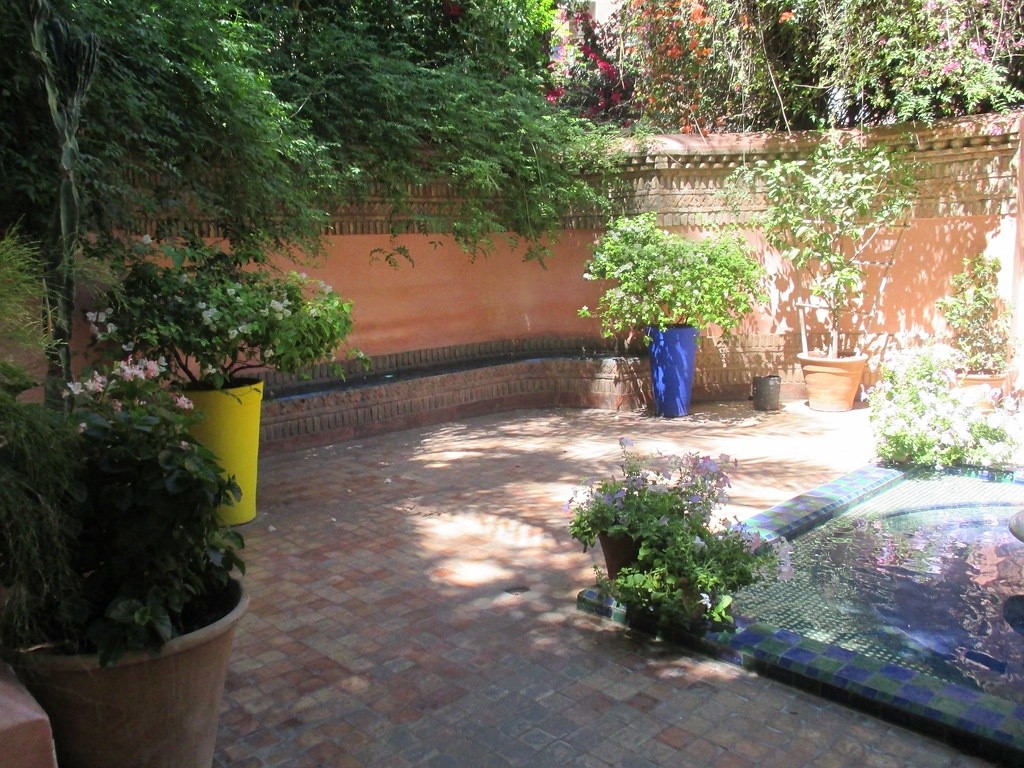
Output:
[{"left": 753, "top": 375, "right": 780, "bottom": 411}]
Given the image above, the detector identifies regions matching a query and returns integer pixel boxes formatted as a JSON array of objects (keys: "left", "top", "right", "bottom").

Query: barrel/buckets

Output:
[{"left": 753, "top": 375, "right": 781, "bottom": 410}]
